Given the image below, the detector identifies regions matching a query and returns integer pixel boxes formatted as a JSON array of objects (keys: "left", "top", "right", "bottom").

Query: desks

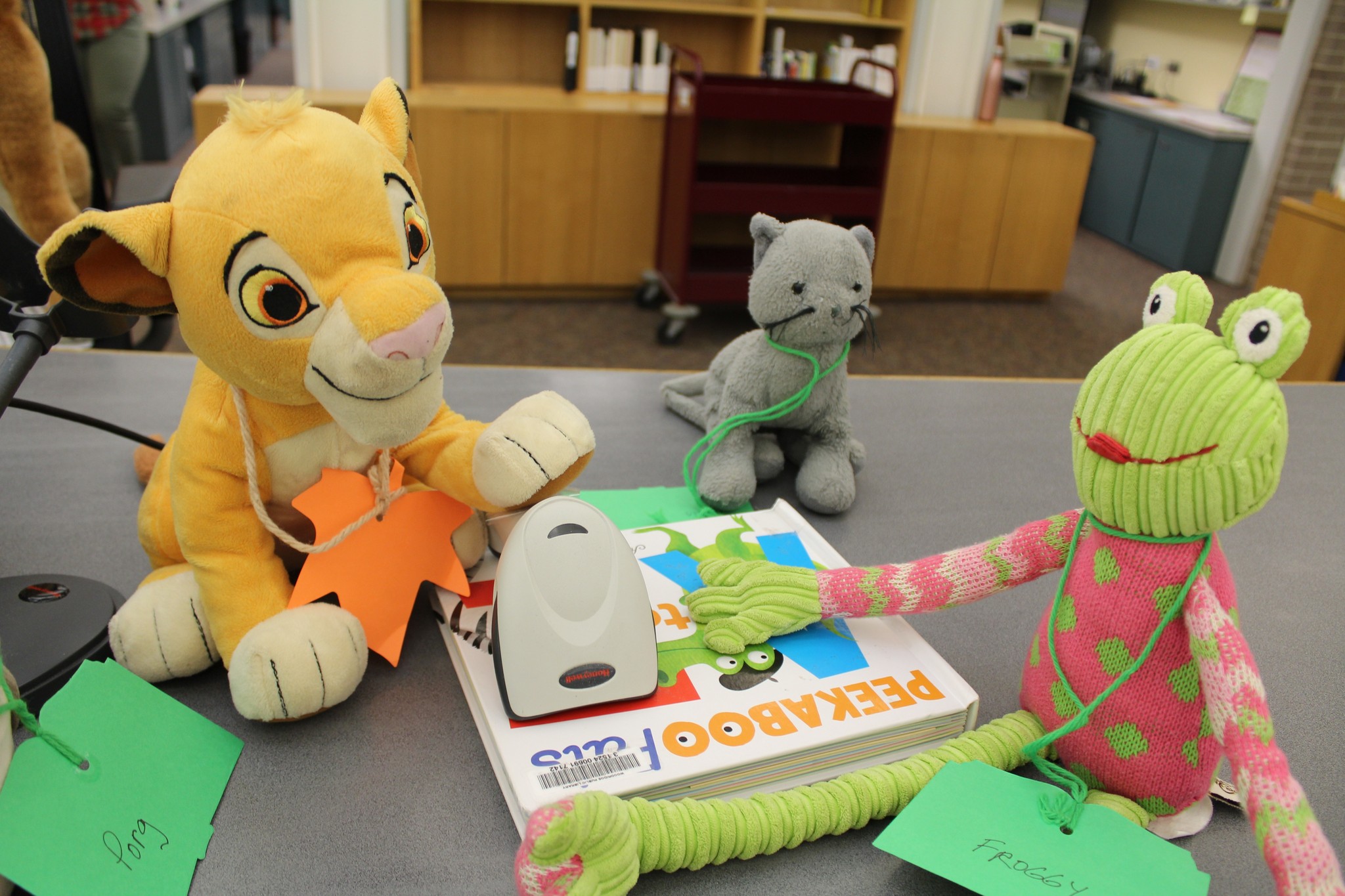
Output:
[{"left": 0, "top": 342, "right": 1345, "bottom": 896}]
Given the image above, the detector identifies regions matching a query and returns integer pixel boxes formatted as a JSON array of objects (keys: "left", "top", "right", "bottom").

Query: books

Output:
[{"left": 431, "top": 488, "right": 978, "bottom": 840}]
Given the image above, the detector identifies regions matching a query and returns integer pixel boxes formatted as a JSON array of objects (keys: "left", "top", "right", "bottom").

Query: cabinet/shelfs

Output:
[
  {"left": 410, "top": 0, "right": 915, "bottom": 113},
  {"left": 190, "top": 84, "right": 671, "bottom": 299},
  {"left": 1065, "top": 96, "right": 1251, "bottom": 276},
  {"left": 1248, "top": 195, "right": 1345, "bottom": 381},
  {"left": 871, "top": 116, "right": 1096, "bottom": 302}
]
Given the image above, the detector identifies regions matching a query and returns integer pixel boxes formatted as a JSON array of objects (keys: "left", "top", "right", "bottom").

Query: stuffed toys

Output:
[
  {"left": 0, "top": 1, "right": 91, "bottom": 245},
  {"left": 660, "top": 212, "right": 875, "bottom": 516},
  {"left": 35, "top": 78, "right": 595, "bottom": 723},
  {"left": 512, "top": 273, "right": 1344, "bottom": 896}
]
